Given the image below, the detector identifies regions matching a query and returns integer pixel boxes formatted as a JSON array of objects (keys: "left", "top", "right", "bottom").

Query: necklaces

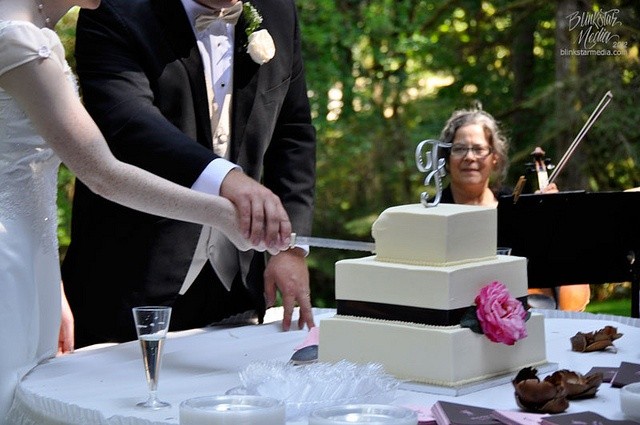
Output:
[{"left": 36, "top": 0, "right": 59, "bottom": 34}]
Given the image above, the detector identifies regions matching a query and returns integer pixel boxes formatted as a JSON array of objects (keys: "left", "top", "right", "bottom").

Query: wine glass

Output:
[{"left": 131, "top": 306, "right": 170, "bottom": 408}]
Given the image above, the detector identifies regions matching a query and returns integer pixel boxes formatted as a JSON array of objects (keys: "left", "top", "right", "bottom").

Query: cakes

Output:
[{"left": 320, "top": 203, "right": 548, "bottom": 386}]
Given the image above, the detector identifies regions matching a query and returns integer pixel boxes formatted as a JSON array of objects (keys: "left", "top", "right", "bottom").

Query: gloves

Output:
[{"left": 35, "top": 104, "right": 292, "bottom": 255}]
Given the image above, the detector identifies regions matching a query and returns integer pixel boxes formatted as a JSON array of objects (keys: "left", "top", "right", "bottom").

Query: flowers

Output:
[{"left": 239, "top": 2, "right": 277, "bottom": 66}]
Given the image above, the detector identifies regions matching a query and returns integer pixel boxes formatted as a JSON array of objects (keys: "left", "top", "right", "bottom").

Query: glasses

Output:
[{"left": 452, "top": 143, "right": 494, "bottom": 159}]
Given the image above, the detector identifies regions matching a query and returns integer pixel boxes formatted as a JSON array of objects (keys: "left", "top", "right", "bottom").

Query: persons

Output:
[
  {"left": 62, "top": 0, "right": 318, "bottom": 347},
  {"left": 0, "top": 0, "right": 292, "bottom": 422},
  {"left": 425, "top": 108, "right": 559, "bottom": 207}
]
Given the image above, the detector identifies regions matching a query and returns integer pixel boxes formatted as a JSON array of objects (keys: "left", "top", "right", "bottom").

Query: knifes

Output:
[{"left": 289, "top": 233, "right": 376, "bottom": 252}]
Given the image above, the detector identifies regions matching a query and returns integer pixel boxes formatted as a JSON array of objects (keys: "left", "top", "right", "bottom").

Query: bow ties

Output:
[{"left": 196, "top": 1, "right": 243, "bottom": 31}]
